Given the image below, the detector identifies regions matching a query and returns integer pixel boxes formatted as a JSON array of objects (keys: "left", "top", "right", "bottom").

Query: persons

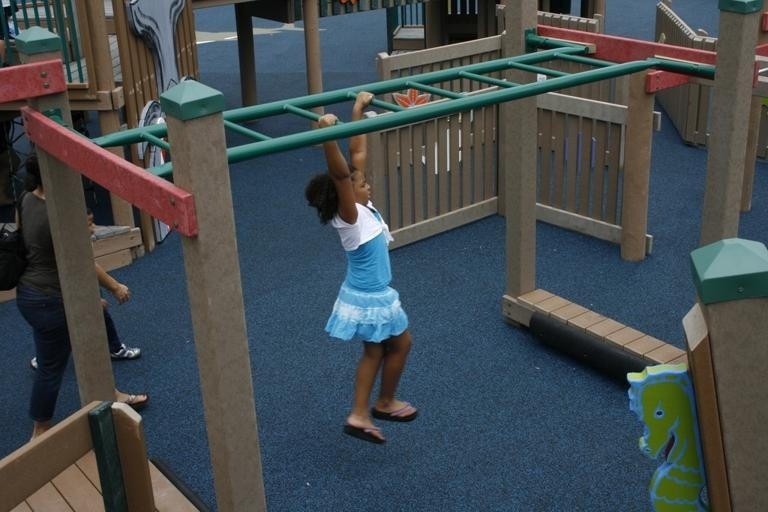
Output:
[
  {"left": 307, "top": 91, "right": 418, "bottom": 441},
  {"left": 0, "top": 0, "right": 21, "bottom": 67},
  {"left": 15, "top": 148, "right": 150, "bottom": 441}
]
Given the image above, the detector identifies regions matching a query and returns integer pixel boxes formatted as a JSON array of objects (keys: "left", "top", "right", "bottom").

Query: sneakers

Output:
[
  {"left": 30, "top": 357, "right": 38, "bottom": 369},
  {"left": 110, "top": 342, "right": 141, "bottom": 359}
]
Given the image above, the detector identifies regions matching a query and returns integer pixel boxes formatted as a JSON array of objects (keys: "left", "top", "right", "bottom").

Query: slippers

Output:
[
  {"left": 120, "top": 392, "right": 149, "bottom": 408},
  {"left": 371, "top": 400, "right": 419, "bottom": 422},
  {"left": 343, "top": 424, "right": 386, "bottom": 445}
]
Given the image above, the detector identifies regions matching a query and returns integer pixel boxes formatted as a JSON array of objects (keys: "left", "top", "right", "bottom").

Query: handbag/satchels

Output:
[{"left": 0, "top": 224, "right": 26, "bottom": 291}]
